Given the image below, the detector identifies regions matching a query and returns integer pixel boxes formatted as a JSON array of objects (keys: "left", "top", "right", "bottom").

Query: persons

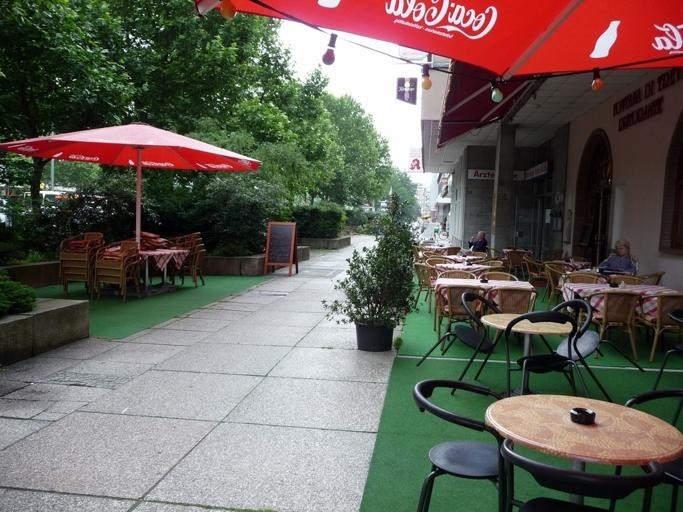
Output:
[
  {"left": 468, "top": 230, "right": 488, "bottom": 253},
  {"left": 599, "top": 239, "right": 640, "bottom": 276}
]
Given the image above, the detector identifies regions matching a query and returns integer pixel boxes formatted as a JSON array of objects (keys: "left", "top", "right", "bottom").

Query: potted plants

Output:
[{"left": 320, "top": 234, "right": 420, "bottom": 352}]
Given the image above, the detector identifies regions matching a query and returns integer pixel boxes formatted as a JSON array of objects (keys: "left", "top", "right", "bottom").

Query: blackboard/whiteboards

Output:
[{"left": 265, "top": 222, "right": 296, "bottom": 266}]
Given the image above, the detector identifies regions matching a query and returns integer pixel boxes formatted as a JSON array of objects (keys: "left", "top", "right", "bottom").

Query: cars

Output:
[{"left": 0, "top": 180, "right": 110, "bottom": 231}]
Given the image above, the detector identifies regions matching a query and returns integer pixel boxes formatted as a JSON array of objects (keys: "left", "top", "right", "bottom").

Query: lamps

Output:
[
  {"left": 319, "top": 33, "right": 607, "bottom": 102},
  {"left": 549, "top": 191, "right": 564, "bottom": 232}
]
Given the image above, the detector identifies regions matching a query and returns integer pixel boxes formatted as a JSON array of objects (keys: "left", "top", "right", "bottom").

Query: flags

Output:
[
  {"left": 395, "top": 76, "right": 417, "bottom": 105},
  {"left": 408, "top": 151, "right": 422, "bottom": 173}
]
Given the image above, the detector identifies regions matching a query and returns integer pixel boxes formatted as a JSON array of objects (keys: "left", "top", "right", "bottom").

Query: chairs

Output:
[{"left": 58, "top": 230, "right": 207, "bottom": 303}]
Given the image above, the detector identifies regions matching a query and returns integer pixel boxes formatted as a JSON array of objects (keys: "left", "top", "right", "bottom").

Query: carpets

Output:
[
  {"left": 25, "top": 275, "right": 277, "bottom": 340},
  {"left": 356, "top": 273, "right": 681, "bottom": 510}
]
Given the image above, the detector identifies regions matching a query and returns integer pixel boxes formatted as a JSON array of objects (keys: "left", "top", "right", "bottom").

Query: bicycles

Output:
[{"left": 438, "top": 228, "right": 448, "bottom": 241}]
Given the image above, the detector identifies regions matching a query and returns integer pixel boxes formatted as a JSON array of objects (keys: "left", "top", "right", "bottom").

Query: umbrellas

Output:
[
  {"left": 0, "top": 119, "right": 264, "bottom": 252},
  {"left": 193, "top": 0, "right": 683, "bottom": 87}
]
